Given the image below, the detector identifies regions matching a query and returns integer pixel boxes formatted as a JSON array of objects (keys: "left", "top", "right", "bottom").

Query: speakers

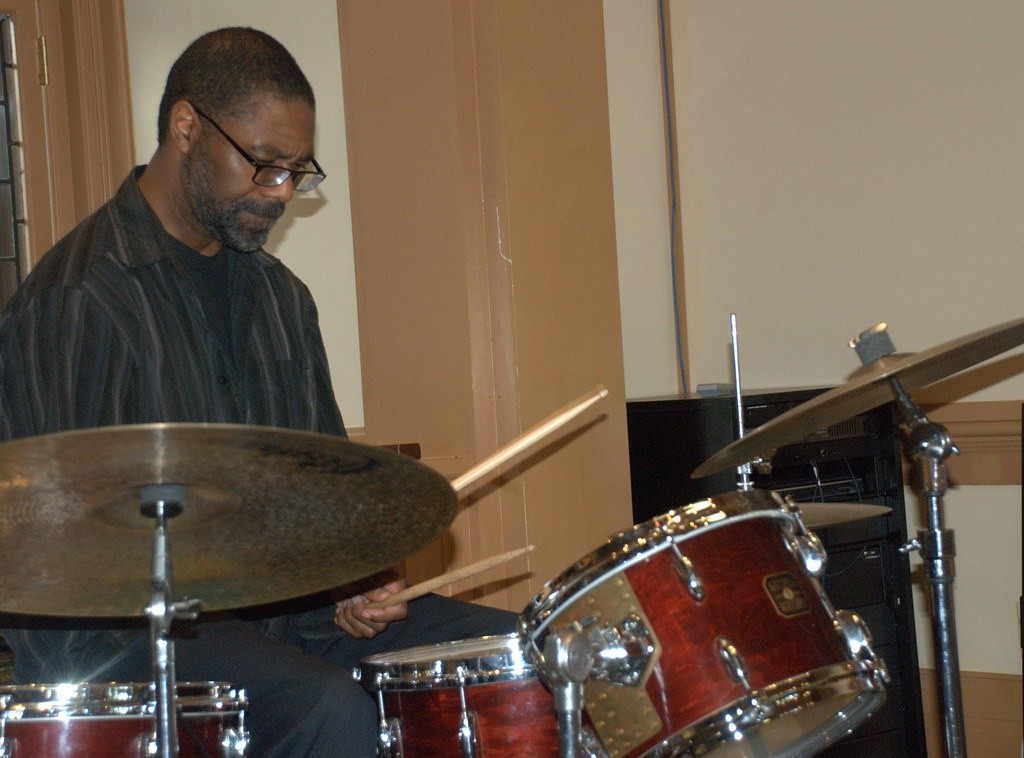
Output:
[{"left": 625, "top": 385, "right": 928, "bottom": 758}]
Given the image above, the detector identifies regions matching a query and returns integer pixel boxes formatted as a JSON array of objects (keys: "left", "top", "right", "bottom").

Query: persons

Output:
[{"left": 0, "top": 23, "right": 529, "bottom": 758}]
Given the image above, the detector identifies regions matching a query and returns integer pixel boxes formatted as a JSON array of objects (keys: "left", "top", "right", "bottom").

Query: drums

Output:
[
  {"left": 363, "top": 635, "right": 561, "bottom": 757},
  {"left": 1, "top": 679, "right": 248, "bottom": 757},
  {"left": 518, "top": 495, "right": 894, "bottom": 757},
  {"left": 796, "top": 498, "right": 899, "bottom": 642}
]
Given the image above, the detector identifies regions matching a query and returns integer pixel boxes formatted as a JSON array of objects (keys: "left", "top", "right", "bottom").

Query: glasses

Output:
[{"left": 188, "top": 101, "right": 326, "bottom": 193}]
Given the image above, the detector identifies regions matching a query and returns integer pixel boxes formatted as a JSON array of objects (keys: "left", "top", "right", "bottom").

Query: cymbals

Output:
[
  {"left": 690, "top": 315, "right": 1024, "bottom": 478},
  {"left": 1, "top": 422, "right": 461, "bottom": 615}
]
